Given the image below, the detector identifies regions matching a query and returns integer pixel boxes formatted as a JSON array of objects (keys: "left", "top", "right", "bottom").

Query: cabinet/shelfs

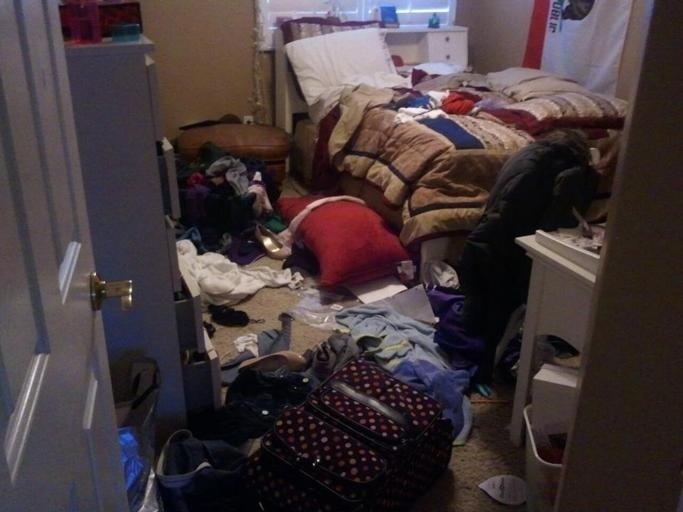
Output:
[{"left": 61, "top": 34, "right": 217, "bottom": 436}]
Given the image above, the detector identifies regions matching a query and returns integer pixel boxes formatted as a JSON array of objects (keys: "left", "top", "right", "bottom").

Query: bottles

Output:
[{"left": 79, "top": 0, "right": 103, "bottom": 45}]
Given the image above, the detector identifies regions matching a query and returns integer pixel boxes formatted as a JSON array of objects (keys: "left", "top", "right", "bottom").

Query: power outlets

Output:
[{"left": 243, "top": 114, "right": 254, "bottom": 125}]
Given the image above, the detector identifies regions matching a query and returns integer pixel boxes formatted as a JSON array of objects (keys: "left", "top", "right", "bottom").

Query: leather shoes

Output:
[
  {"left": 255, "top": 223, "right": 292, "bottom": 260},
  {"left": 238, "top": 351, "right": 307, "bottom": 372}
]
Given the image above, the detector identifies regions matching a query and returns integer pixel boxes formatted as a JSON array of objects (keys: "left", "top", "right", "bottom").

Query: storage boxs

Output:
[{"left": 523, "top": 402, "right": 576, "bottom": 512}]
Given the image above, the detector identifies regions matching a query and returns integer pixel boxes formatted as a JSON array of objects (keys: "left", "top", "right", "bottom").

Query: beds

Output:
[{"left": 275, "top": 24, "right": 629, "bottom": 287}]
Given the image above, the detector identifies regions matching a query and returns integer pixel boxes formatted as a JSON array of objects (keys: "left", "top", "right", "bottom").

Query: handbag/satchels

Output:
[{"left": 244, "top": 355, "right": 453, "bottom": 511}]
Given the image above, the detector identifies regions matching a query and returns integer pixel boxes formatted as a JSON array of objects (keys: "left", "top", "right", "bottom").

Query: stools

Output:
[{"left": 178, "top": 123, "right": 292, "bottom": 181}]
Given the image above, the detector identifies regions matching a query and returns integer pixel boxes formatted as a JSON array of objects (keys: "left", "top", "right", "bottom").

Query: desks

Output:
[{"left": 513, "top": 221, "right": 605, "bottom": 449}]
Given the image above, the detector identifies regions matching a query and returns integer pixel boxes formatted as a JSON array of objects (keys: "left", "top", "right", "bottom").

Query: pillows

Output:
[
  {"left": 280, "top": 16, "right": 382, "bottom": 70},
  {"left": 499, "top": 92, "right": 629, "bottom": 137},
  {"left": 277, "top": 193, "right": 409, "bottom": 290},
  {"left": 280, "top": 28, "right": 402, "bottom": 105}
]
